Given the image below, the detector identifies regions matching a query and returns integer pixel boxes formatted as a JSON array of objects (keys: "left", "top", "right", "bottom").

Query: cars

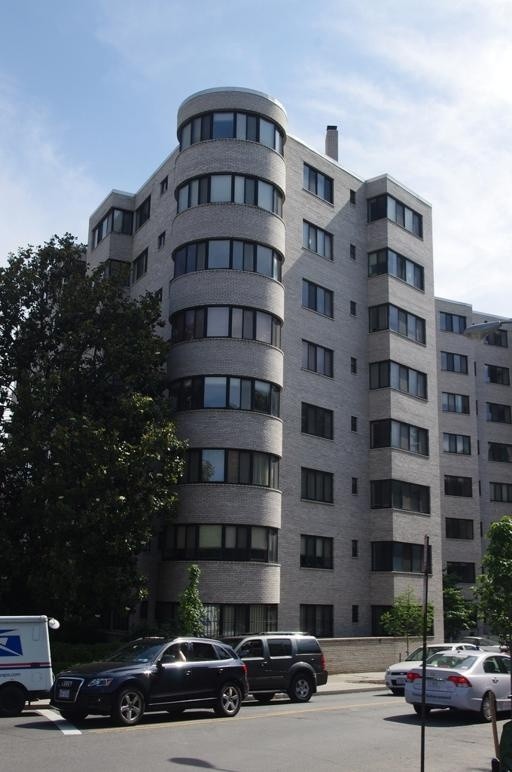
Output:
[
  {"left": 383, "top": 636, "right": 512, "bottom": 722},
  {"left": 50, "top": 630, "right": 329, "bottom": 724}
]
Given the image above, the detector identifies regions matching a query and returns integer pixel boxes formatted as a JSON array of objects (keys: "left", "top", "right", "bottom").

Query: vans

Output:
[{"left": 0, "top": 615, "right": 60, "bottom": 715}]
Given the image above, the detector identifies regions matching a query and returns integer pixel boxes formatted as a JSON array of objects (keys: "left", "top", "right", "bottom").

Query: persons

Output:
[{"left": 169, "top": 643, "right": 186, "bottom": 662}]
